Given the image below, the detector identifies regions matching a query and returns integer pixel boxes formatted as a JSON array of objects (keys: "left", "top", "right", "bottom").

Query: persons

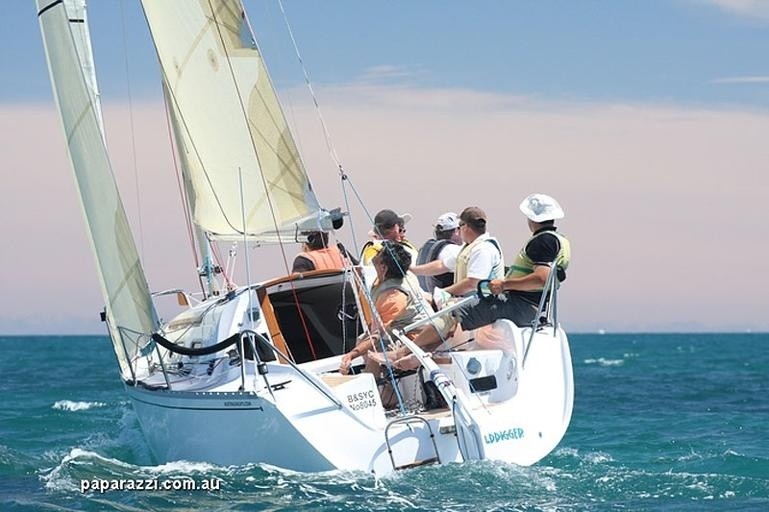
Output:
[
  {"left": 293, "top": 232, "right": 349, "bottom": 274},
  {"left": 368, "top": 193, "right": 571, "bottom": 371},
  {"left": 338, "top": 206, "right": 504, "bottom": 411}
]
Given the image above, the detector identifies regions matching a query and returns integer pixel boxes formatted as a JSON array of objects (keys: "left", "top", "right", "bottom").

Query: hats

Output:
[
  {"left": 374, "top": 208, "right": 412, "bottom": 224},
  {"left": 435, "top": 206, "right": 487, "bottom": 232},
  {"left": 519, "top": 193, "right": 564, "bottom": 223}
]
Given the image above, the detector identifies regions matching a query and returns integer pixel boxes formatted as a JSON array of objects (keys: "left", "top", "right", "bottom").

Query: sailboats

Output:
[{"left": 35, "top": 0, "right": 574, "bottom": 475}]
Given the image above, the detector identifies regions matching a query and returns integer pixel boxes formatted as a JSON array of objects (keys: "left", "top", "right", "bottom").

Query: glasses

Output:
[{"left": 400, "top": 229, "right": 406, "bottom": 233}]
